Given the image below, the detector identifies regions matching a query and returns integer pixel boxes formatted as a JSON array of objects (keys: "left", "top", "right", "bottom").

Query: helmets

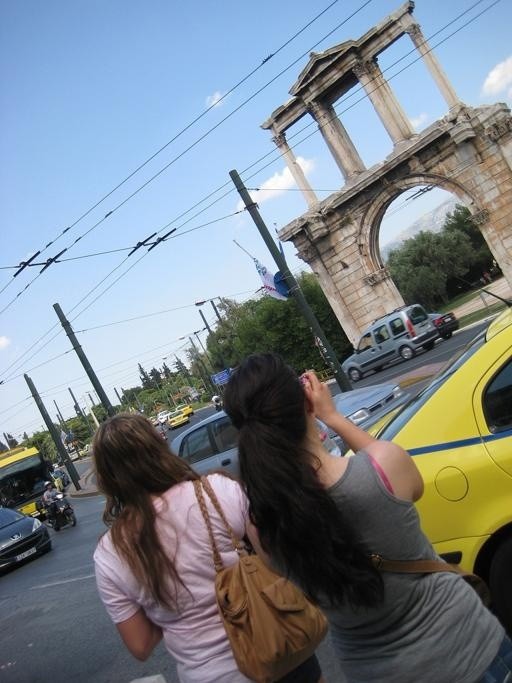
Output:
[{"left": 44, "top": 481, "right": 51, "bottom": 486}]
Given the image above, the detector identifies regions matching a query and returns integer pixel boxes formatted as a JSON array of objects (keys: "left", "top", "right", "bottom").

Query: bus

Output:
[{"left": 0, "top": 444, "right": 64, "bottom": 522}]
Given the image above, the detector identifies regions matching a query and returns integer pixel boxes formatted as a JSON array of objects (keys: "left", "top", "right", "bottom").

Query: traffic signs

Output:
[{"left": 210, "top": 369, "right": 230, "bottom": 383}]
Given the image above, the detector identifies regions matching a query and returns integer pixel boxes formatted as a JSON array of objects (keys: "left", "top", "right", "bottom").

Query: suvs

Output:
[{"left": 341, "top": 304, "right": 440, "bottom": 381}]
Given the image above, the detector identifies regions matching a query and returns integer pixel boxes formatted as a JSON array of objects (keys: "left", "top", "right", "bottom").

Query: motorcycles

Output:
[
  {"left": 214, "top": 397, "right": 221, "bottom": 411},
  {"left": 34, "top": 490, "right": 76, "bottom": 531}
]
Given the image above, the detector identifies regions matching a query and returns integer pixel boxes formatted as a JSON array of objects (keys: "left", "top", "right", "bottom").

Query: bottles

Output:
[{"left": 316, "top": 418, "right": 341, "bottom": 460}]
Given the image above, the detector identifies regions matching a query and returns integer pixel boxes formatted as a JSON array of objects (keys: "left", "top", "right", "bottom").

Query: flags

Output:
[{"left": 253, "top": 257, "right": 287, "bottom": 301}]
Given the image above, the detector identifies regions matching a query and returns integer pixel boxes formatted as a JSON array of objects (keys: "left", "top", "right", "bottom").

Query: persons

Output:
[
  {"left": 222, "top": 346, "right": 511, "bottom": 683},
  {"left": 89, "top": 409, "right": 326, "bottom": 681},
  {"left": 31, "top": 475, "right": 48, "bottom": 494},
  {"left": 42, "top": 480, "right": 66, "bottom": 524}
]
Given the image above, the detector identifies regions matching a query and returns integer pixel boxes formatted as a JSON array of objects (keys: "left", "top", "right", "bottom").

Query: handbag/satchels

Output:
[{"left": 214, "top": 555, "right": 331, "bottom": 683}]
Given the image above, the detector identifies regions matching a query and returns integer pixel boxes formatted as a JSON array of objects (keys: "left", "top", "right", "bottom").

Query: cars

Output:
[
  {"left": 53, "top": 467, "right": 69, "bottom": 486},
  {"left": 357, "top": 305, "right": 512, "bottom": 637},
  {"left": 149, "top": 404, "right": 194, "bottom": 429},
  {"left": 0, "top": 506, "right": 52, "bottom": 576},
  {"left": 170, "top": 382, "right": 411, "bottom": 496},
  {"left": 428, "top": 311, "right": 460, "bottom": 339}
]
